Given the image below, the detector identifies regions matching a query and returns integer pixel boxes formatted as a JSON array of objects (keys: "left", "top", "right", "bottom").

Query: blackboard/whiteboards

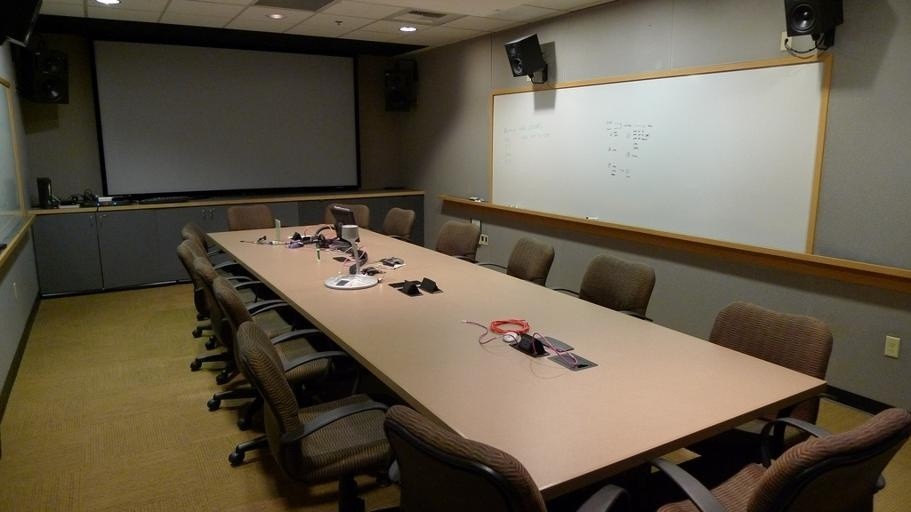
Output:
[{"left": 487, "top": 50, "right": 833, "bottom": 255}]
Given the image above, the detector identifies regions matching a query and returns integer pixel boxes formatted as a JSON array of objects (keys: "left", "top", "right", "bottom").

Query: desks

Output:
[{"left": 205, "top": 223, "right": 826, "bottom": 505}]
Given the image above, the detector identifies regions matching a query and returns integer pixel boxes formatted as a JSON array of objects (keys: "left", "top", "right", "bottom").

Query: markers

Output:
[{"left": 586, "top": 216, "right": 598, "bottom": 220}]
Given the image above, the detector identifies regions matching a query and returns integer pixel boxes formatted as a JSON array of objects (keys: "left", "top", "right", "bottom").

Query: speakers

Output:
[
  {"left": 385, "top": 67, "right": 414, "bottom": 112},
  {"left": 504, "top": 33, "right": 544, "bottom": 78},
  {"left": 785, "top": 0, "right": 843, "bottom": 37},
  {"left": 21, "top": 50, "right": 70, "bottom": 105}
]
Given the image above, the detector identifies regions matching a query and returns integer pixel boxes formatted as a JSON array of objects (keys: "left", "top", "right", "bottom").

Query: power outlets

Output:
[{"left": 884, "top": 335, "right": 900, "bottom": 357}]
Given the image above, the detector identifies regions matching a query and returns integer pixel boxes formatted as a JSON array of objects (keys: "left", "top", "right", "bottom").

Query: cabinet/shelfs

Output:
[
  {"left": 32, "top": 208, "right": 161, "bottom": 300},
  {"left": 162, "top": 202, "right": 300, "bottom": 287}
]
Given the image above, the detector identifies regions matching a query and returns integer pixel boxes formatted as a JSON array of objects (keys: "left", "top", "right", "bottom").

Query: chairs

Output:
[
  {"left": 706, "top": 301, "right": 832, "bottom": 460},
  {"left": 382, "top": 404, "right": 625, "bottom": 512},
  {"left": 649, "top": 404, "right": 911, "bottom": 511},
  {"left": 175, "top": 202, "right": 659, "bottom": 428},
  {"left": 235, "top": 321, "right": 396, "bottom": 512}
]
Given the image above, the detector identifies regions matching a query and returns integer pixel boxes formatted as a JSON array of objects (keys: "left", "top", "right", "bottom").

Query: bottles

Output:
[{"left": 52, "top": 198, "right": 58, "bottom": 208}]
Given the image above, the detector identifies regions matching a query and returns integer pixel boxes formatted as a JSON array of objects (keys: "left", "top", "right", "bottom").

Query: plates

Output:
[{"left": 324, "top": 274, "right": 379, "bottom": 291}]
